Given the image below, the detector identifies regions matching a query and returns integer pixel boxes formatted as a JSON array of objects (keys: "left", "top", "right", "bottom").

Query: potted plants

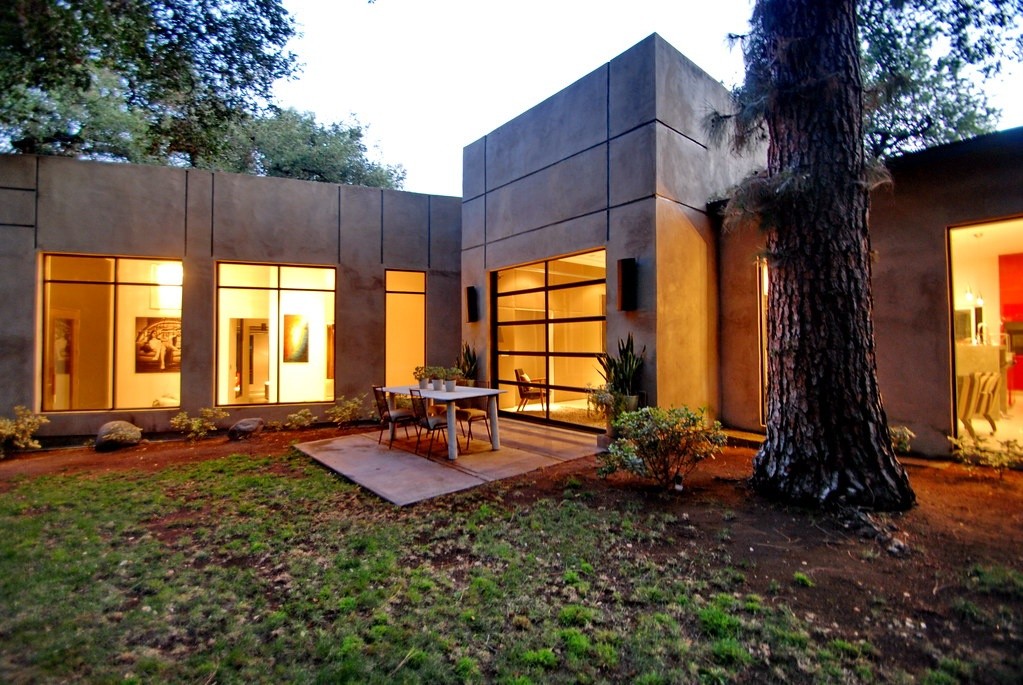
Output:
[
  {"left": 427, "top": 366, "right": 445, "bottom": 390},
  {"left": 439, "top": 367, "right": 463, "bottom": 392},
  {"left": 593, "top": 332, "right": 646, "bottom": 436},
  {"left": 414, "top": 367, "right": 429, "bottom": 389}
]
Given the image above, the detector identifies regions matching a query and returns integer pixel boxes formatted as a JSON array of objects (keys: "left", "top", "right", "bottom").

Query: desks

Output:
[{"left": 376, "top": 384, "right": 508, "bottom": 459}]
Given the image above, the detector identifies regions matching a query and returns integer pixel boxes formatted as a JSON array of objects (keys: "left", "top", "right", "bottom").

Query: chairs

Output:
[
  {"left": 372, "top": 385, "right": 421, "bottom": 449},
  {"left": 409, "top": 388, "right": 462, "bottom": 460},
  {"left": 514, "top": 368, "right": 546, "bottom": 414},
  {"left": 456, "top": 381, "right": 492, "bottom": 450},
  {"left": 957, "top": 371, "right": 1001, "bottom": 442},
  {"left": 435, "top": 379, "right": 476, "bottom": 444}
]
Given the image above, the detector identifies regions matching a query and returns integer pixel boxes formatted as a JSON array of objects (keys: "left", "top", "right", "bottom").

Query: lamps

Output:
[{"left": 965, "top": 284, "right": 984, "bottom": 306}]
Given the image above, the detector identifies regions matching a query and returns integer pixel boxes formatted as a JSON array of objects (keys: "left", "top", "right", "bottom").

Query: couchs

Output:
[{"left": 136, "top": 342, "right": 169, "bottom": 366}]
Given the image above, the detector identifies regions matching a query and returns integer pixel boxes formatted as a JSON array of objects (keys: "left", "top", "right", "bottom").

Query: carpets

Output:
[{"left": 517, "top": 404, "right": 607, "bottom": 428}]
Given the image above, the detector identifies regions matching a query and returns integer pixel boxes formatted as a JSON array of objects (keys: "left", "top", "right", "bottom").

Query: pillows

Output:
[{"left": 521, "top": 373, "right": 533, "bottom": 391}]
